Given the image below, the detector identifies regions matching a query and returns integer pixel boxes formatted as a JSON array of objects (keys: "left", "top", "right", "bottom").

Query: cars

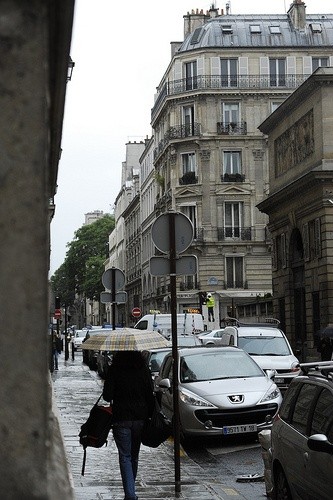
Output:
[
  {"left": 73, "top": 330, "right": 90, "bottom": 351},
  {"left": 265, "top": 363, "right": 332, "bottom": 500},
  {"left": 82, "top": 330, "right": 111, "bottom": 367},
  {"left": 153, "top": 346, "right": 283, "bottom": 435},
  {"left": 196, "top": 328, "right": 224, "bottom": 346},
  {"left": 95, "top": 349, "right": 117, "bottom": 376},
  {"left": 143, "top": 347, "right": 179, "bottom": 388}
]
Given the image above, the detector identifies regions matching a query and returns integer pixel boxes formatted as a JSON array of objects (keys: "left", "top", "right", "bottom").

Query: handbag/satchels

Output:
[{"left": 140, "top": 394, "right": 174, "bottom": 448}]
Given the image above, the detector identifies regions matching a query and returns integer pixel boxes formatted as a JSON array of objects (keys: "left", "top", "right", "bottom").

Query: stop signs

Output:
[
  {"left": 55, "top": 310, "right": 61, "bottom": 319},
  {"left": 131, "top": 308, "right": 142, "bottom": 317}
]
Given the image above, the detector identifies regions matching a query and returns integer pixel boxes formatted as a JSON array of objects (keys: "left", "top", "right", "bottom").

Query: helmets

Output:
[{"left": 207, "top": 293, "right": 211, "bottom": 297}]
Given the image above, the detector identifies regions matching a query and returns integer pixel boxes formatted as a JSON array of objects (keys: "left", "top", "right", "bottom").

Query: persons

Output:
[
  {"left": 66, "top": 326, "right": 90, "bottom": 344},
  {"left": 103, "top": 351, "right": 154, "bottom": 500},
  {"left": 50, "top": 330, "right": 61, "bottom": 373},
  {"left": 202, "top": 293, "right": 215, "bottom": 322},
  {"left": 317, "top": 336, "right": 333, "bottom": 376}
]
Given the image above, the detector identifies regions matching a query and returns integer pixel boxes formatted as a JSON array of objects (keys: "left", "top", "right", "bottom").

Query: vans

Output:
[
  {"left": 222, "top": 325, "right": 301, "bottom": 391},
  {"left": 134, "top": 313, "right": 205, "bottom": 342}
]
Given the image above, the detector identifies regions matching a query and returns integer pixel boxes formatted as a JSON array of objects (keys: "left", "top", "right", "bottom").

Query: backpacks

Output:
[{"left": 78, "top": 393, "right": 113, "bottom": 477}]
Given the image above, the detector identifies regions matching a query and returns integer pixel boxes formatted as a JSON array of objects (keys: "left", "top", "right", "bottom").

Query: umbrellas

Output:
[
  {"left": 79, "top": 329, "right": 170, "bottom": 352},
  {"left": 314, "top": 325, "right": 333, "bottom": 336}
]
